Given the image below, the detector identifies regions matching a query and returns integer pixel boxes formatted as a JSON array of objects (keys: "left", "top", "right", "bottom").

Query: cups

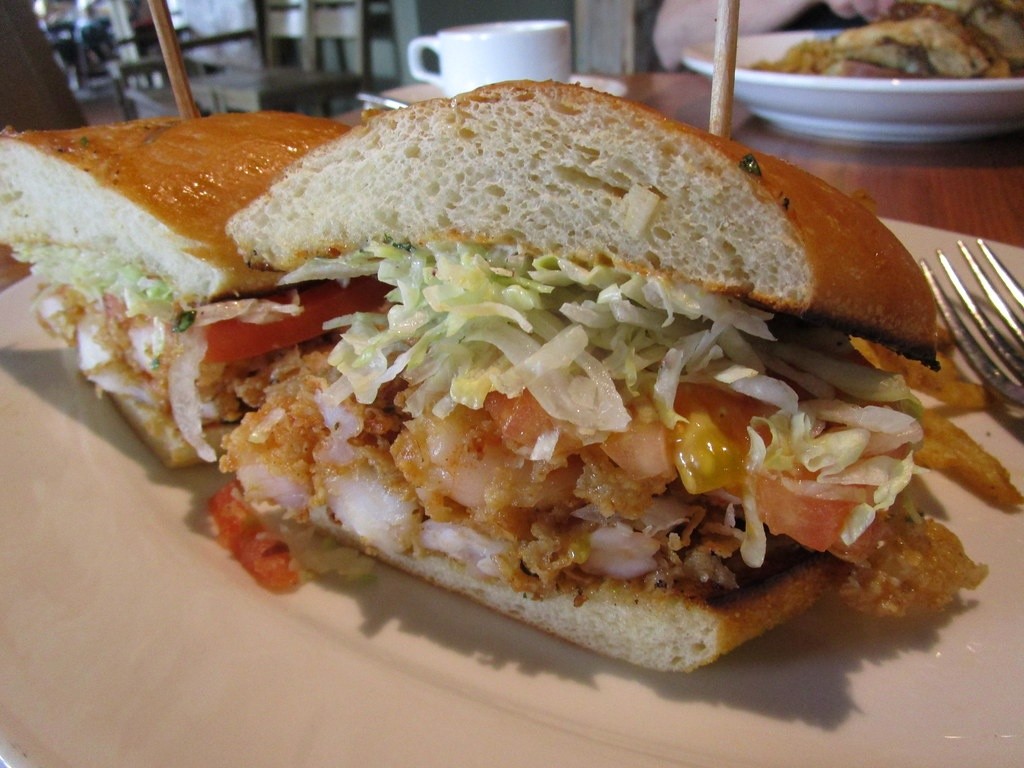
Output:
[{"left": 408, "top": 20, "right": 571, "bottom": 98}]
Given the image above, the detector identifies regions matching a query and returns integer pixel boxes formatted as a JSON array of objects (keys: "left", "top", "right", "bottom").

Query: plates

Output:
[
  {"left": 378, "top": 75, "right": 628, "bottom": 98},
  {"left": 682, "top": 28, "right": 1024, "bottom": 143}
]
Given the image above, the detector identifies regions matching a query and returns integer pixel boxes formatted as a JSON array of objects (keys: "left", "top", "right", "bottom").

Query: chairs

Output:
[{"left": 107, "top": 0, "right": 374, "bottom": 126}]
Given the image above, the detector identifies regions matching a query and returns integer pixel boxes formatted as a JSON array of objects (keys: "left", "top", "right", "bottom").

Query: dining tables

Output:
[{"left": 0, "top": 70, "right": 1024, "bottom": 768}]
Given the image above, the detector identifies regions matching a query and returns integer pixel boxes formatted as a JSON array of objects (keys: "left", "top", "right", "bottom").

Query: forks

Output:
[{"left": 919, "top": 238, "right": 1024, "bottom": 407}]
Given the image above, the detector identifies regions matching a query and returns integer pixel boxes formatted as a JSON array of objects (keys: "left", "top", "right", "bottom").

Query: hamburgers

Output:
[
  {"left": 221, "top": 81, "right": 989, "bottom": 676},
  {"left": 0, "top": 111, "right": 383, "bottom": 471}
]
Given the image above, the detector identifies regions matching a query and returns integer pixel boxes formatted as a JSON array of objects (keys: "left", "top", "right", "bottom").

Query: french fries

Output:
[{"left": 847, "top": 324, "right": 1024, "bottom": 510}]
